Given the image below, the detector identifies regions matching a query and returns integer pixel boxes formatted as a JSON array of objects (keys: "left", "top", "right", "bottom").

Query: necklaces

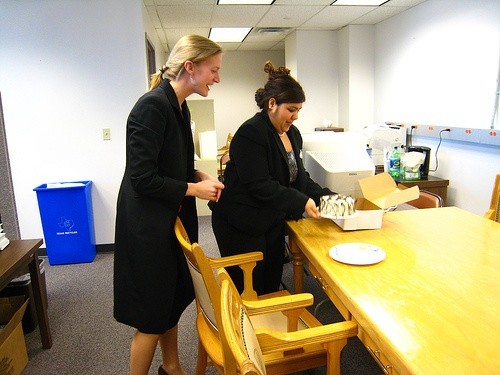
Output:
[{"left": 279, "top": 132, "right": 285, "bottom": 136}]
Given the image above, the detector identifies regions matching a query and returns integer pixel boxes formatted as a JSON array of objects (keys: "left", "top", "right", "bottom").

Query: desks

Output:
[
  {"left": 286, "top": 206, "right": 500, "bottom": 375},
  {"left": 0, "top": 239, "right": 53, "bottom": 349},
  {"left": 194, "top": 157, "right": 217, "bottom": 216},
  {"left": 375, "top": 171, "right": 449, "bottom": 205}
]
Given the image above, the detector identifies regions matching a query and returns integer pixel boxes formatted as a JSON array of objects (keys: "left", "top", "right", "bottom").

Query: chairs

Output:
[{"left": 175, "top": 216, "right": 358, "bottom": 375}]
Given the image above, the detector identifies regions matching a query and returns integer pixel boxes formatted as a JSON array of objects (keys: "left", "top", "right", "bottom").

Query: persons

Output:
[
  {"left": 113, "top": 34, "right": 225, "bottom": 375},
  {"left": 208, "top": 60, "right": 338, "bottom": 296}
]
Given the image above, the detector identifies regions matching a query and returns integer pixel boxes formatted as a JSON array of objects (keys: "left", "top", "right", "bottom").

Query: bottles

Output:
[{"left": 389, "top": 147, "right": 400, "bottom": 177}]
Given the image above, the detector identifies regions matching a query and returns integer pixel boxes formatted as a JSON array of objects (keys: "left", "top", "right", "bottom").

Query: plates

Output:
[{"left": 329, "top": 243, "right": 385, "bottom": 266}]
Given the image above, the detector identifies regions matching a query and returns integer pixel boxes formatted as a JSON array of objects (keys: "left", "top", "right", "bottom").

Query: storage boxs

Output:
[
  {"left": 333, "top": 172, "right": 419, "bottom": 231},
  {"left": 0, "top": 296, "right": 31, "bottom": 375}
]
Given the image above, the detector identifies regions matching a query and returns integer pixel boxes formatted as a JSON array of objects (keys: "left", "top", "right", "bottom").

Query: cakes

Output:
[{"left": 319, "top": 194, "right": 356, "bottom": 218}]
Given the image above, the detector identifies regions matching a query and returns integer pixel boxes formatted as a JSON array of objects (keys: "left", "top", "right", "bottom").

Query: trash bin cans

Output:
[
  {"left": 32, "top": 180, "right": 96, "bottom": 265},
  {"left": 12, "top": 258, "right": 48, "bottom": 310}
]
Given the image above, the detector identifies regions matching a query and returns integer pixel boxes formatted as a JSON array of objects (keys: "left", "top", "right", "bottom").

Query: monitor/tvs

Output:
[{"left": 301, "top": 131, "right": 376, "bottom": 200}]
